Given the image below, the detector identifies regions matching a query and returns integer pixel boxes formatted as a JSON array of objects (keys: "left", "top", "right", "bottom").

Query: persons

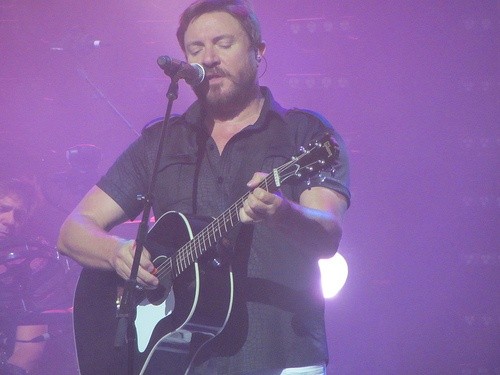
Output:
[
  {"left": 56, "top": 0, "right": 352, "bottom": 375},
  {"left": 0, "top": 175, "right": 80, "bottom": 375}
]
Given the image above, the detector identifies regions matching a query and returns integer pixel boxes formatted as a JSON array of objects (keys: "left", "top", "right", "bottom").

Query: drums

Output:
[{"left": 0, "top": 238, "right": 71, "bottom": 314}]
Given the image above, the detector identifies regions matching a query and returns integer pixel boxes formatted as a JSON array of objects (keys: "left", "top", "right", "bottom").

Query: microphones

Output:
[
  {"left": 156, "top": 55, "right": 205, "bottom": 87},
  {"left": 93, "top": 38, "right": 115, "bottom": 50}
]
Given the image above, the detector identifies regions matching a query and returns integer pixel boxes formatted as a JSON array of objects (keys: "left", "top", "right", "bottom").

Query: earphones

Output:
[{"left": 256, "top": 54, "right": 262, "bottom": 63}]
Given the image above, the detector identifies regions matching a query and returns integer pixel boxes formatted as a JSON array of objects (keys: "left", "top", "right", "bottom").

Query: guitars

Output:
[{"left": 72, "top": 130, "right": 339, "bottom": 375}]
